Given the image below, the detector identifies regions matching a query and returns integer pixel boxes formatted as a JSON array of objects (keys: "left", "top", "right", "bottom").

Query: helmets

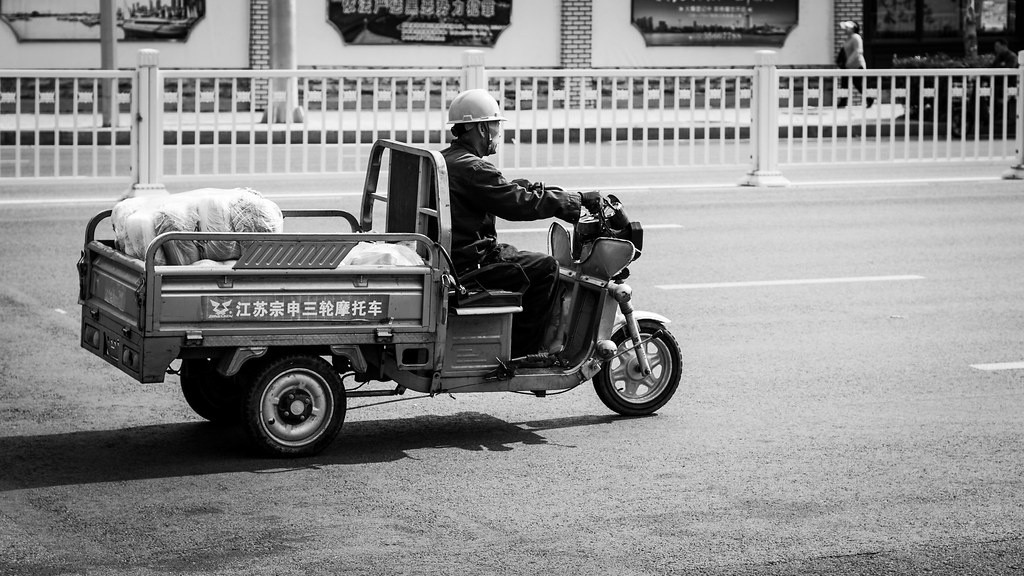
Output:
[{"left": 447, "top": 89, "right": 508, "bottom": 124}]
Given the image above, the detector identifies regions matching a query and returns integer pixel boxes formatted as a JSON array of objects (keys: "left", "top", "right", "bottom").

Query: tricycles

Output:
[{"left": 75, "top": 140, "right": 682, "bottom": 458}]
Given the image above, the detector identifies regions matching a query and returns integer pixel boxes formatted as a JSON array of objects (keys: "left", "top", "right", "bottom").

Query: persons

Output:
[
  {"left": 991, "top": 39, "right": 1018, "bottom": 69},
  {"left": 836, "top": 21, "right": 875, "bottom": 108},
  {"left": 428, "top": 88, "right": 603, "bottom": 369}
]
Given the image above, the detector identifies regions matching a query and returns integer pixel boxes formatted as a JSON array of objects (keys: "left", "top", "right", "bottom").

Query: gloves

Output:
[
  {"left": 511, "top": 178, "right": 532, "bottom": 191},
  {"left": 577, "top": 191, "right": 600, "bottom": 214}
]
